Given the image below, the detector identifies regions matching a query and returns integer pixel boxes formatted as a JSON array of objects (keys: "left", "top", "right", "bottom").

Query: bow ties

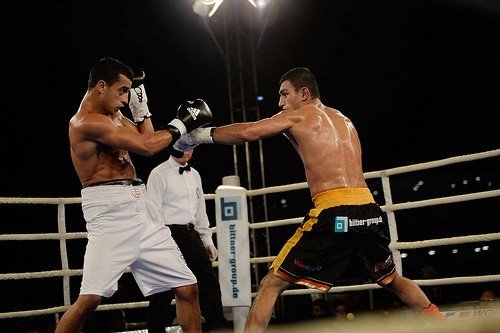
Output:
[{"left": 180, "top": 165, "right": 190, "bottom": 174}]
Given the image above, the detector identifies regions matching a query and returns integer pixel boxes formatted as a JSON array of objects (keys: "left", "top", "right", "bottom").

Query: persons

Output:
[
  {"left": 334, "top": 298, "right": 348, "bottom": 320},
  {"left": 479, "top": 290, "right": 497, "bottom": 308},
  {"left": 309, "top": 298, "right": 332, "bottom": 320},
  {"left": 170, "top": 67, "right": 448, "bottom": 333},
  {"left": 54, "top": 58, "right": 226, "bottom": 333}
]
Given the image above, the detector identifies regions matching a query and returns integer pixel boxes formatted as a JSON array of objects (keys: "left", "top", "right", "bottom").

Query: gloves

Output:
[
  {"left": 128, "top": 71, "right": 152, "bottom": 122},
  {"left": 169, "top": 123, "right": 214, "bottom": 158},
  {"left": 168, "top": 99, "right": 213, "bottom": 141}
]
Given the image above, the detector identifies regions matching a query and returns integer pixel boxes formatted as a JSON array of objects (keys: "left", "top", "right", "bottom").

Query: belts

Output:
[{"left": 168, "top": 225, "right": 194, "bottom": 231}]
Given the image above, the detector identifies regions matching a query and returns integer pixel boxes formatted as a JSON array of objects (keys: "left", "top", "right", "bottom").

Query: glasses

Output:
[{"left": 481, "top": 297, "right": 495, "bottom": 303}]
